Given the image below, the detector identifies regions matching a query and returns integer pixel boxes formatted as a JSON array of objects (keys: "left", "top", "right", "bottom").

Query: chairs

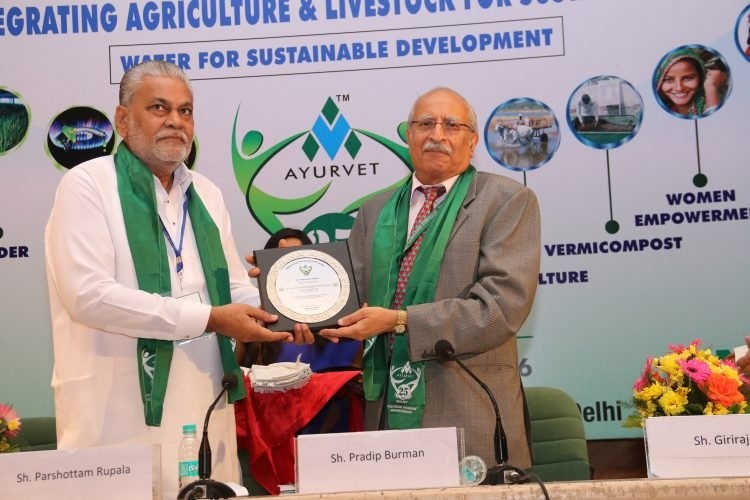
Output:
[
  {"left": 522, "top": 386, "right": 591, "bottom": 481},
  {"left": 7, "top": 417, "right": 57, "bottom": 452}
]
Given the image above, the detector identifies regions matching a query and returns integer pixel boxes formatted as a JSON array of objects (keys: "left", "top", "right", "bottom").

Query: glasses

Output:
[{"left": 407, "top": 119, "right": 474, "bottom": 136}]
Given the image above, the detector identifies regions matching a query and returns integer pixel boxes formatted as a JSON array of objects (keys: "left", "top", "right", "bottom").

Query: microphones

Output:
[
  {"left": 435, "top": 340, "right": 531, "bottom": 486},
  {"left": 176, "top": 372, "right": 239, "bottom": 500}
]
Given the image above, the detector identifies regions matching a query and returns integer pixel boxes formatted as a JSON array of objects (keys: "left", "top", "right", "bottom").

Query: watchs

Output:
[{"left": 393, "top": 310, "right": 407, "bottom": 335}]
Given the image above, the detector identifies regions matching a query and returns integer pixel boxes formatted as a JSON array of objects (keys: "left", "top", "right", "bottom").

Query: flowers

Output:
[
  {"left": 0, "top": 403, "right": 22, "bottom": 453},
  {"left": 622, "top": 338, "right": 750, "bottom": 428}
]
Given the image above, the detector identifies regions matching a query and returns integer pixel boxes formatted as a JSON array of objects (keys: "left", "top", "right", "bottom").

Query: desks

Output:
[{"left": 229, "top": 477, "right": 750, "bottom": 500}]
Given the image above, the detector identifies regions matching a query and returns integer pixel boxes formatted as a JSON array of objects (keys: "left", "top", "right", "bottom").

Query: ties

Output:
[{"left": 388, "top": 186, "right": 445, "bottom": 358}]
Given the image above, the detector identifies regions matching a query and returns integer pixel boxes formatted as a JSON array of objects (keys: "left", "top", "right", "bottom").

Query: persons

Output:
[
  {"left": 245, "top": 87, "right": 541, "bottom": 485},
  {"left": 653, "top": 50, "right": 728, "bottom": 117},
  {"left": 515, "top": 114, "right": 525, "bottom": 126},
  {"left": 576, "top": 93, "right": 599, "bottom": 129},
  {"left": 235, "top": 227, "right": 364, "bottom": 434},
  {"left": 45, "top": 61, "right": 339, "bottom": 500}
]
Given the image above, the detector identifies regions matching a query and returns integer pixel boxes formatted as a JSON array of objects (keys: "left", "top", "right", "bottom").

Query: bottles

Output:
[
  {"left": 465, "top": 455, "right": 488, "bottom": 485},
  {"left": 178, "top": 424, "right": 200, "bottom": 492}
]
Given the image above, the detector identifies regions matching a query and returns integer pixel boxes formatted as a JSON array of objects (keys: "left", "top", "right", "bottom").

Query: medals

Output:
[{"left": 254, "top": 240, "right": 363, "bottom": 333}]
